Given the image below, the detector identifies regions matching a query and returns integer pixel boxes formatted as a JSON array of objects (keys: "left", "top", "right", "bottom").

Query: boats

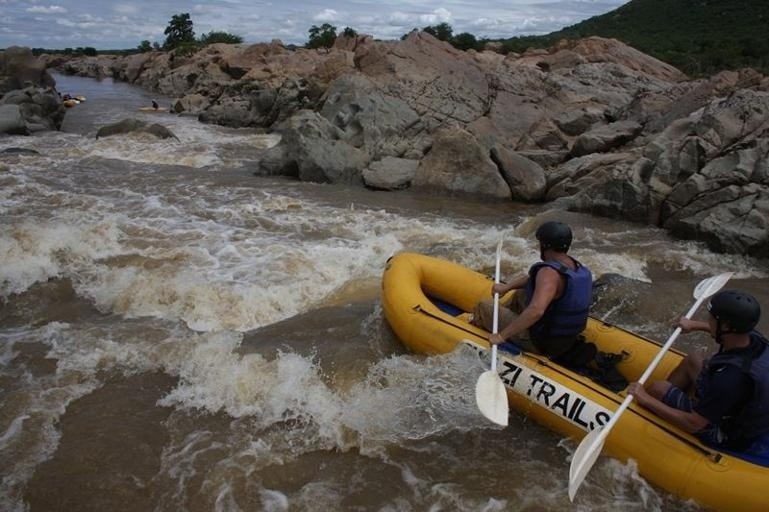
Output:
[
  {"left": 380, "top": 252, "right": 769, "bottom": 512},
  {"left": 138, "top": 106, "right": 169, "bottom": 112},
  {"left": 63, "top": 95, "right": 87, "bottom": 107}
]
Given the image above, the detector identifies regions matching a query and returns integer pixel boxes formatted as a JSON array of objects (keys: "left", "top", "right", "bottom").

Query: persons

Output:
[
  {"left": 152, "top": 101, "right": 158, "bottom": 109},
  {"left": 627, "top": 291, "right": 769, "bottom": 454},
  {"left": 58, "top": 92, "right": 82, "bottom": 101},
  {"left": 469, "top": 222, "right": 593, "bottom": 359}
]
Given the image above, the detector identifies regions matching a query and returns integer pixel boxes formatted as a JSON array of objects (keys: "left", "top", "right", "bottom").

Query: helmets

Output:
[
  {"left": 707, "top": 290, "right": 760, "bottom": 334},
  {"left": 536, "top": 221, "right": 572, "bottom": 251}
]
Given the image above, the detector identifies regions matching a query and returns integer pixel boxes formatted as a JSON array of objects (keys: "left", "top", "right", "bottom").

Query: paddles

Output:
[
  {"left": 476, "top": 234, "right": 509, "bottom": 427},
  {"left": 568, "top": 272, "right": 735, "bottom": 502}
]
